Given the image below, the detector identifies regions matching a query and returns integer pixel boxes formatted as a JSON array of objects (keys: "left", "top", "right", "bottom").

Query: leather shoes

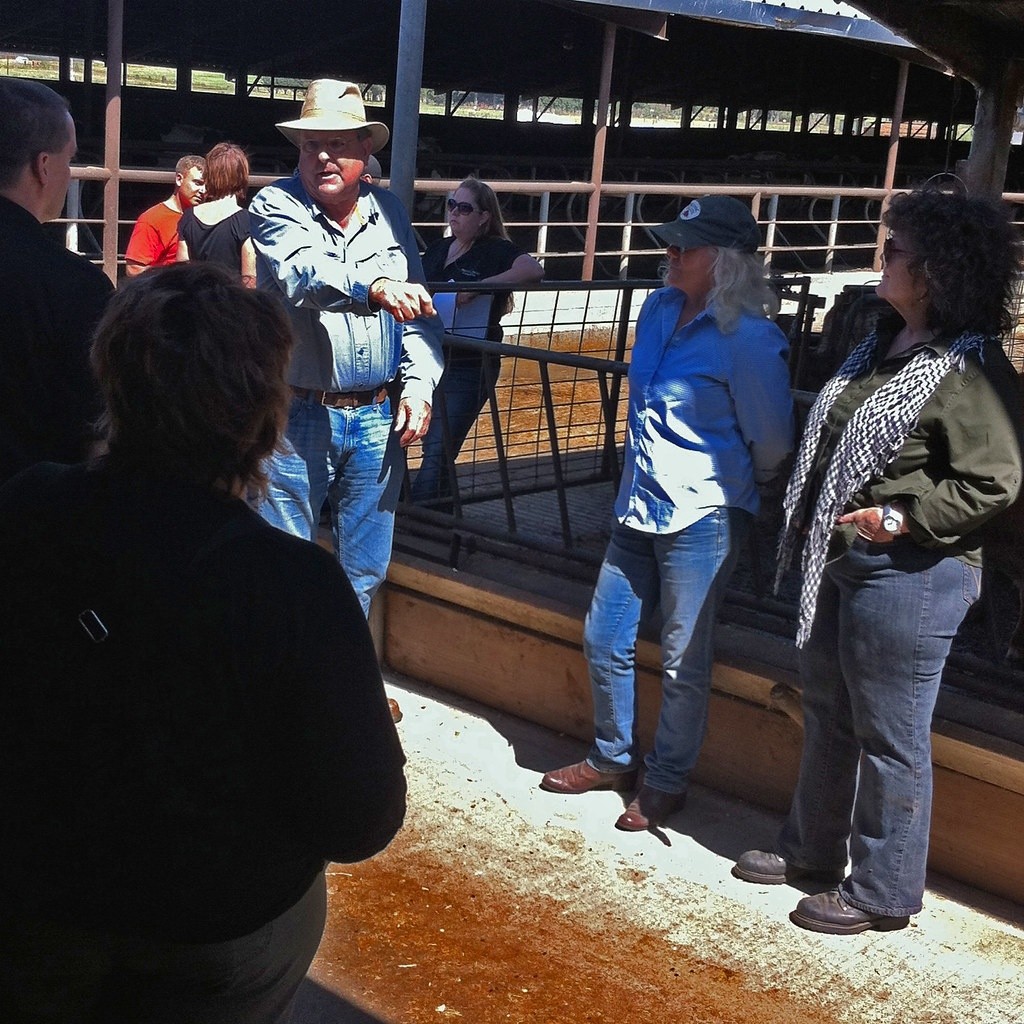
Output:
[
  {"left": 733, "top": 850, "right": 848, "bottom": 885},
  {"left": 790, "top": 889, "right": 911, "bottom": 934},
  {"left": 542, "top": 759, "right": 638, "bottom": 794},
  {"left": 616, "top": 784, "right": 688, "bottom": 829}
]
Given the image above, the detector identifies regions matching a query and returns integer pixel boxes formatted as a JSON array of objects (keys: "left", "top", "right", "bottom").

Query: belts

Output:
[{"left": 286, "top": 384, "right": 389, "bottom": 408}]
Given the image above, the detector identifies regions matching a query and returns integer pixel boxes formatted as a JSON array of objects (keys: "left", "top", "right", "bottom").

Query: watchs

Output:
[{"left": 883, "top": 503, "right": 902, "bottom": 535}]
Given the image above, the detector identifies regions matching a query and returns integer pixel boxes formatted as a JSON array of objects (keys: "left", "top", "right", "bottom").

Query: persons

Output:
[
  {"left": 176, "top": 143, "right": 256, "bottom": 289},
  {"left": 411, "top": 180, "right": 544, "bottom": 515},
  {"left": 734, "top": 193, "right": 1023, "bottom": 934},
  {"left": 1, "top": 76, "right": 117, "bottom": 487},
  {"left": 246, "top": 78, "right": 446, "bottom": 722},
  {"left": 125, "top": 156, "right": 208, "bottom": 277},
  {"left": 0, "top": 265, "right": 407, "bottom": 1022},
  {"left": 541, "top": 196, "right": 792, "bottom": 830}
]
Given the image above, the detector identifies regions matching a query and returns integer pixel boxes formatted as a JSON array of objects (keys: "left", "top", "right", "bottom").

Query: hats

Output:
[
  {"left": 275, "top": 79, "right": 390, "bottom": 155},
  {"left": 648, "top": 195, "right": 761, "bottom": 255}
]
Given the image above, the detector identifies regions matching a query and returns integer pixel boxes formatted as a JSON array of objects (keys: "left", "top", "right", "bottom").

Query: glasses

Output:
[
  {"left": 883, "top": 239, "right": 917, "bottom": 263},
  {"left": 298, "top": 137, "right": 362, "bottom": 154},
  {"left": 446, "top": 199, "right": 484, "bottom": 215}
]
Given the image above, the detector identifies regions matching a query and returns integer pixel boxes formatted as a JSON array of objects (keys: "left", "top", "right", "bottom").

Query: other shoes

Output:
[{"left": 386, "top": 698, "right": 402, "bottom": 723}]
